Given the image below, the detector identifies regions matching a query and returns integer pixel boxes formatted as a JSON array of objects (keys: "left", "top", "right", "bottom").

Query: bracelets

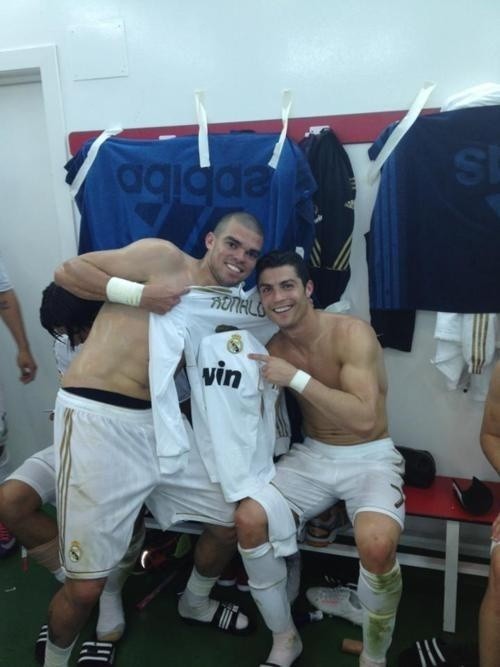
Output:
[
  {"left": 105, "top": 276, "right": 145, "bottom": 307},
  {"left": 287, "top": 369, "right": 312, "bottom": 394}
]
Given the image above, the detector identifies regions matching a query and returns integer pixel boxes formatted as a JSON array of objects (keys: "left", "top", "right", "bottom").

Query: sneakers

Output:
[
  {"left": 128, "top": 532, "right": 180, "bottom": 576},
  {"left": 216, "top": 560, "right": 250, "bottom": 592},
  {"left": 305, "top": 559, "right": 366, "bottom": 627},
  {"left": 305, "top": 509, "right": 350, "bottom": 547}
]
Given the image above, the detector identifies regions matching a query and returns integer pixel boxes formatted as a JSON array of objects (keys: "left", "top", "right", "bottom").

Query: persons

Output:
[
  {"left": 232, "top": 249, "right": 408, "bottom": 666},
  {"left": 43, "top": 209, "right": 264, "bottom": 665},
  {"left": 0, "top": 280, "right": 148, "bottom": 644},
  {"left": 475, "top": 356, "right": 500, "bottom": 667},
  {"left": 1, "top": 261, "right": 38, "bottom": 559}
]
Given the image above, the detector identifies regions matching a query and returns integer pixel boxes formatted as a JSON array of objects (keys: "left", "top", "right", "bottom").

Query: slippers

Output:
[
  {"left": 396, "top": 636, "right": 479, "bottom": 667},
  {"left": 34, "top": 623, "right": 49, "bottom": 667},
  {"left": 75, "top": 629, "right": 119, "bottom": 667},
  {"left": 258, "top": 661, "right": 283, "bottom": 667},
  {"left": 176, "top": 593, "right": 256, "bottom": 636}
]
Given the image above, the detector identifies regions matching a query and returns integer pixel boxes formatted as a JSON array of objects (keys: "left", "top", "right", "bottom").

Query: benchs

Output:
[{"left": 141, "top": 476, "right": 500, "bottom": 635}]
